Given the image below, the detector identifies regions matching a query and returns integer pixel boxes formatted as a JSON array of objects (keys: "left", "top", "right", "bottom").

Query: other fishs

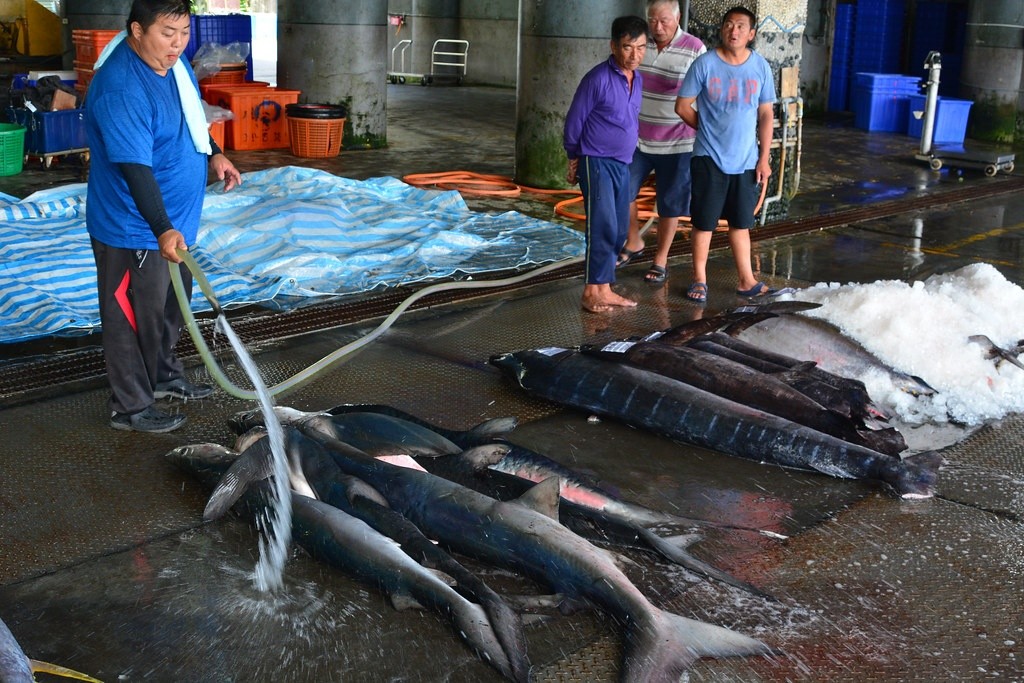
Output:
[{"left": 161, "top": 302, "right": 949, "bottom": 683}]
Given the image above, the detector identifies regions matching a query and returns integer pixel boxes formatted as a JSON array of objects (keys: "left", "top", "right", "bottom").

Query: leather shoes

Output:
[
  {"left": 111, "top": 407, "right": 188, "bottom": 433},
  {"left": 154, "top": 378, "right": 215, "bottom": 400}
]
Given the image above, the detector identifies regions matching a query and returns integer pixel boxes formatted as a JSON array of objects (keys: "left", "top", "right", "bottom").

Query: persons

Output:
[
  {"left": 564, "top": 16, "right": 647, "bottom": 314},
  {"left": 618, "top": 0, "right": 707, "bottom": 283},
  {"left": 85, "top": 0, "right": 241, "bottom": 431},
  {"left": 675, "top": 6, "right": 779, "bottom": 302}
]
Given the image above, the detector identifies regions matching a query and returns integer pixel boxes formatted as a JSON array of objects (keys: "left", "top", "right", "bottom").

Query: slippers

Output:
[
  {"left": 615, "top": 247, "right": 645, "bottom": 268},
  {"left": 736, "top": 281, "right": 776, "bottom": 296},
  {"left": 687, "top": 283, "right": 708, "bottom": 302},
  {"left": 644, "top": 264, "right": 670, "bottom": 282}
]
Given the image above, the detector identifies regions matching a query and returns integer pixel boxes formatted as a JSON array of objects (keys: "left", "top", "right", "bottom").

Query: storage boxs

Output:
[
  {"left": 0, "top": 26, "right": 346, "bottom": 178},
  {"left": 855, "top": 71, "right": 975, "bottom": 143}
]
